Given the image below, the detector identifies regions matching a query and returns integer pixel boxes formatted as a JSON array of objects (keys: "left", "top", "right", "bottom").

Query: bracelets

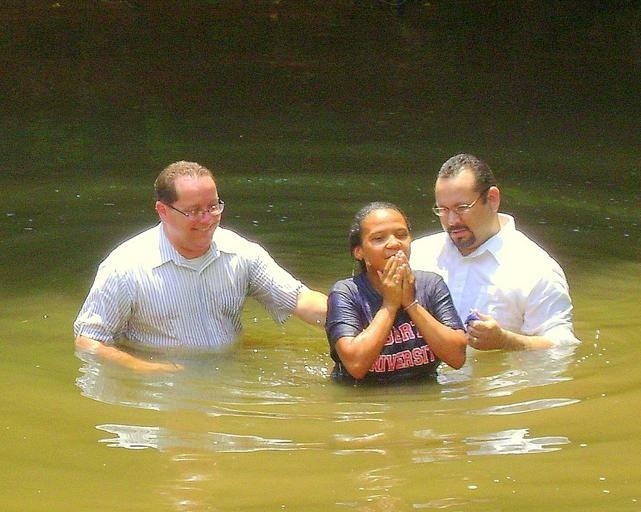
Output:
[{"left": 403, "top": 300, "right": 422, "bottom": 312}]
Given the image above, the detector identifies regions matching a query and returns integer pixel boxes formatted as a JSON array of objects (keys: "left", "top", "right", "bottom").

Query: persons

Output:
[
  {"left": 72, "top": 160, "right": 332, "bottom": 378},
  {"left": 406, "top": 154, "right": 584, "bottom": 355},
  {"left": 323, "top": 200, "right": 471, "bottom": 387}
]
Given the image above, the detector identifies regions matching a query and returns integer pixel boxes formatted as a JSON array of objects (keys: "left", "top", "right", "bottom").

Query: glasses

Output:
[
  {"left": 160, "top": 196, "right": 226, "bottom": 222},
  {"left": 432, "top": 184, "right": 497, "bottom": 218}
]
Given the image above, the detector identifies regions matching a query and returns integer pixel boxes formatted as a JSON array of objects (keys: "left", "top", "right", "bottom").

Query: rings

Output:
[{"left": 473, "top": 336, "right": 479, "bottom": 345}]
